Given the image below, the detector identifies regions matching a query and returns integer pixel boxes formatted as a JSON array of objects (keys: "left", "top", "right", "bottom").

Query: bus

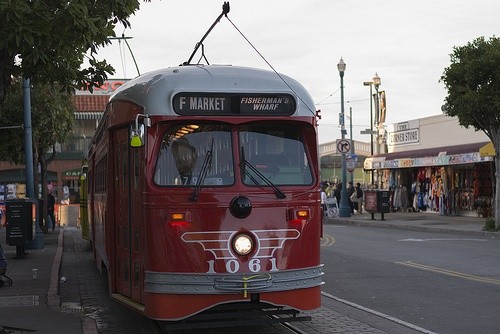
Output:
[{"left": 79, "top": 1, "right": 324, "bottom": 327}]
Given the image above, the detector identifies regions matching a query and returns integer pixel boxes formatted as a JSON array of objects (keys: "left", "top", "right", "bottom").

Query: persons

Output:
[
  {"left": 47, "top": 188, "right": 55, "bottom": 232},
  {"left": 345, "top": 182, "right": 363, "bottom": 217},
  {"left": 319, "top": 180, "right": 343, "bottom": 218}
]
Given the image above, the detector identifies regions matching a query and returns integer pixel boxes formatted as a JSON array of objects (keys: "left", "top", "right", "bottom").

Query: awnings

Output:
[{"left": 363, "top": 140, "right": 497, "bottom": 171}]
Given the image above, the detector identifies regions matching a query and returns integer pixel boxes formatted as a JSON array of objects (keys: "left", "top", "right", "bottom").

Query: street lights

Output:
[
  {"left": 337, "top": 56, "right": 352, "bottom": 218},
  {"left": 373, "top": 72, "right": 381, "bottom": 155}
]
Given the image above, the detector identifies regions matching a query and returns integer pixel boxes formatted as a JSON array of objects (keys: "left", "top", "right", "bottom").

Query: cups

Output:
[{"left": 32, "top": 269, "right": 38, "bottom": 279}]
